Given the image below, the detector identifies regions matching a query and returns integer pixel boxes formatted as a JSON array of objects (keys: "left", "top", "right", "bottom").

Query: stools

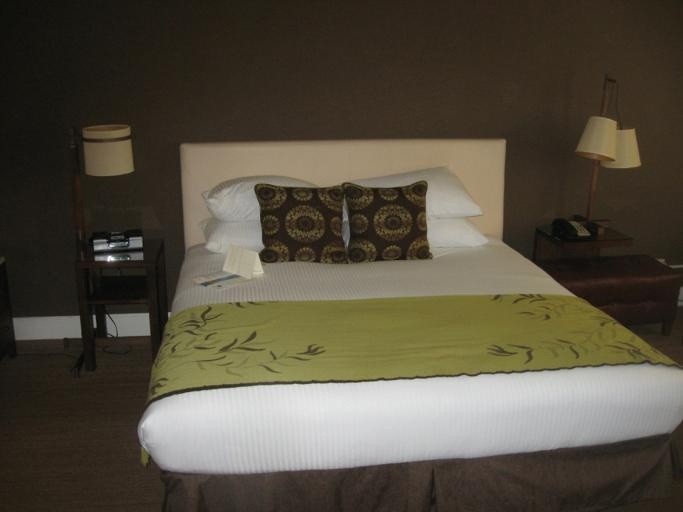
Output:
[{"left": 531, "top": 254, "right": 682, "bottom": 336}]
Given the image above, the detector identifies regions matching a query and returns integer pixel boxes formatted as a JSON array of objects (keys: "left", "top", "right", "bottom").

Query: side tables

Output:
[{"left": 74, "top": 236, "right": 168, "bottom": 370}]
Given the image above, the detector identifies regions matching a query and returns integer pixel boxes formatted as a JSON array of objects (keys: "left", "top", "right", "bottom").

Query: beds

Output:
[{"left": 135, "top": 137, "right": 683, "bottom": 511}]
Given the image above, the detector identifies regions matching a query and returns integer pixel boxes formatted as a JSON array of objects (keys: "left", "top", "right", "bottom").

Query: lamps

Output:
[
  {"left": 570, "top": 76, "right": 643, "bottom": 223},
  {"left": 67, "top": 123, "right": 136, "bottom": 239}
]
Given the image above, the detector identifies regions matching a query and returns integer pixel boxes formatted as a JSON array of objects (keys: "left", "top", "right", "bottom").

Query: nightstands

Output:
[{"left": 533, "top": 225, "right": 633, "bottom": 260}]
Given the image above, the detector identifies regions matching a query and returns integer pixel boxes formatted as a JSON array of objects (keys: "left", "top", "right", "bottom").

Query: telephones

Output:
[{"left": 556, "top": 216, "right": 599, "bottom": 240}]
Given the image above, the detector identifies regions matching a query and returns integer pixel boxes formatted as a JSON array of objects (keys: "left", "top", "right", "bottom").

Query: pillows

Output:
[{"left": 198, "top": 165, "right": 490, "bottom": 266}]
